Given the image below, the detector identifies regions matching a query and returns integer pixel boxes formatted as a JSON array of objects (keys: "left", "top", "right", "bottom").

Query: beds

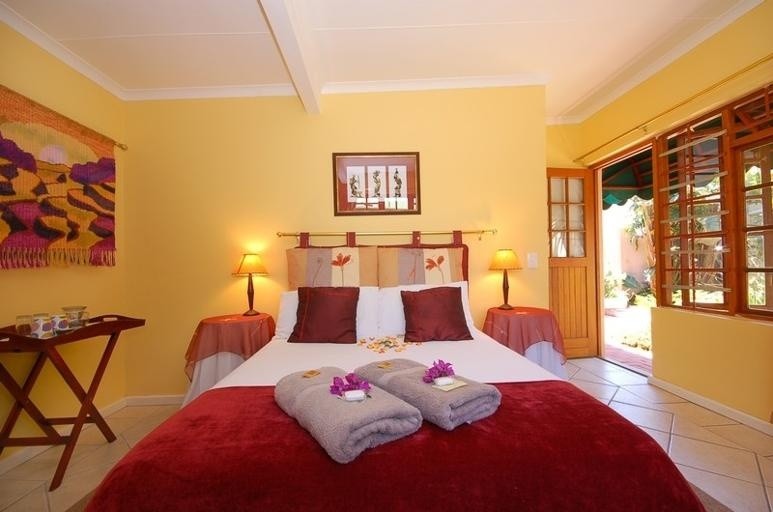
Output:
[{"left": 85, "top": 230, "right": 707, "bottom": 510}]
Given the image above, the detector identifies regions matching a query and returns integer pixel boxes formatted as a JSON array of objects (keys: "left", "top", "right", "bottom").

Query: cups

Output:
[{"left": 16, "top": 306, "right": 89, "bottom": 339}]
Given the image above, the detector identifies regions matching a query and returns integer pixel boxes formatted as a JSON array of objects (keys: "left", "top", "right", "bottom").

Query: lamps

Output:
[
  {"left": 230, "top": 252, "right": 268, "bottom": 318},
  {"left": 488, "top": 247, "right": 522, "bottom": 310}
]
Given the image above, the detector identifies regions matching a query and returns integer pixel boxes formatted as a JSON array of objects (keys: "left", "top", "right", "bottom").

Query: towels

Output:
[{"left": 271, "top": 357, "right": 503, "bottom": 465}]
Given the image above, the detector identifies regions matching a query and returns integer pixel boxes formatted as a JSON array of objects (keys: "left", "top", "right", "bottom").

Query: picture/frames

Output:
[{"left": 331, "top": 152, "right": 421, "bottom": 217}]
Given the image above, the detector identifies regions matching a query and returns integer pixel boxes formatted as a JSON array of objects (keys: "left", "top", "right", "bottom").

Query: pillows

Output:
[{"left": 272, "top": 279, "right": 476, "bottom": 345}]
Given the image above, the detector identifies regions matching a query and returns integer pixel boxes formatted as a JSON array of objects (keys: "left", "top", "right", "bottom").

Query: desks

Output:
[
  {"left": 180, "top": 312, "right": 277, "bottom": 413},
  {"left": 484, "top": 306, "right": 565, "bottom": 371},
  {"left": 0, "top": 313, "right": 147, "bottom": 491}
]
